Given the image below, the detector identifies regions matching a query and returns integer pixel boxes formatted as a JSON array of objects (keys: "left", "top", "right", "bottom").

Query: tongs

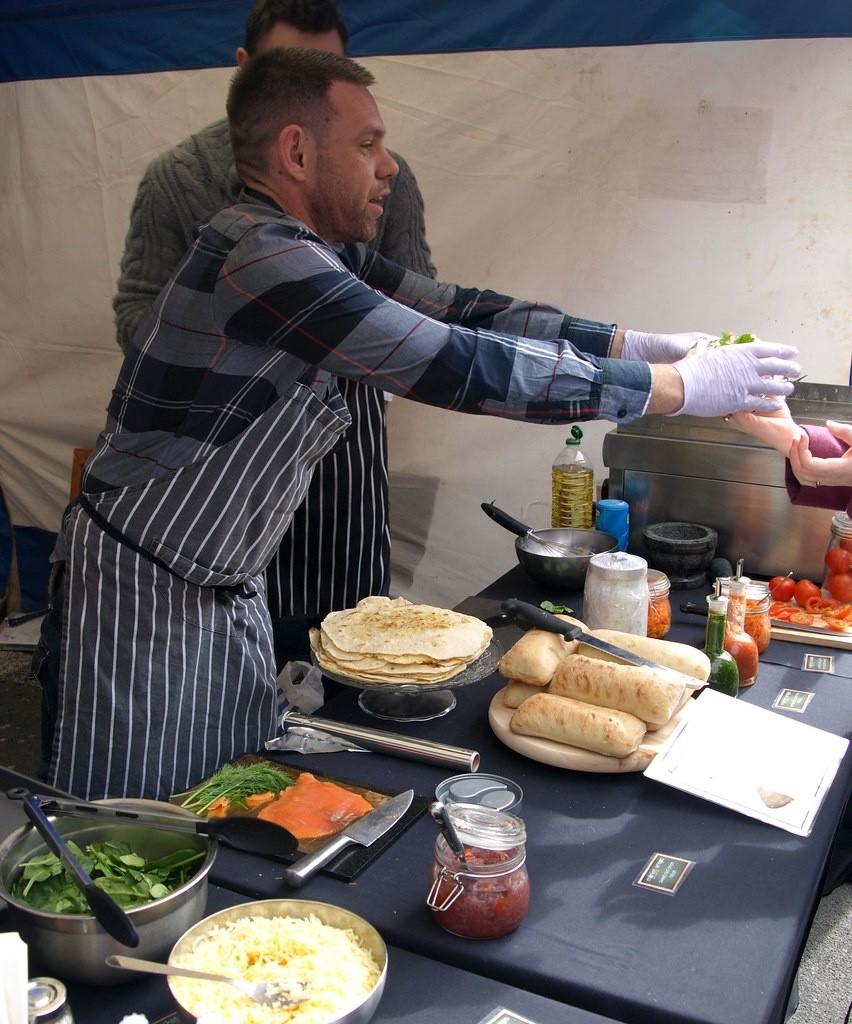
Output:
[{"left": 24, "top": 793, "right": 299, "bottom": 948}]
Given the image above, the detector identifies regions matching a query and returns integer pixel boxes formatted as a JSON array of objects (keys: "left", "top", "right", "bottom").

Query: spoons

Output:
[{"left": 104, "top": 955, "right": 307, "bottom": 1008}]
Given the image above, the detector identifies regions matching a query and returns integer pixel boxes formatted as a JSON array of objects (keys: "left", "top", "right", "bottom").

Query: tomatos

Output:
[
  {"left": 768, "top": 576, "right": 821, "bottom": 607},
  {"left": 823, "top": 533, "right": 852, "bottom": 604},
  {"left": 768, "top": 597, "right": 852, "bottom": 631}
]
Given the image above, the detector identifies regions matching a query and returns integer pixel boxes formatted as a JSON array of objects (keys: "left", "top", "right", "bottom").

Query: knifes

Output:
[
  {"left": 501, "top": 600, "right": 709, "bottom": 686},
  {"left": 680, "top": 601, "right": 852, "bottom": 637},
  {"left": 281, "top": 789, "right": 414, "bottom": 885}
]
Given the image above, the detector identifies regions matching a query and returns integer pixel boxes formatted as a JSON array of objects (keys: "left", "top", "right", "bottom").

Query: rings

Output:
[
  {"left": 815, "top": 481, "right": 821, "bottom": 488},
  {"left": 724, "top": 414, "right": 732, "bottom": 422}
]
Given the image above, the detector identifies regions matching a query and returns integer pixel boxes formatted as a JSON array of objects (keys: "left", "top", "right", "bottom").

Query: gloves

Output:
[
  {"left": 620, "top": 329, "right": 735, "bottom": 364},
  {"left": 665, "top": 338, "right": 802, "bottom": 419}
]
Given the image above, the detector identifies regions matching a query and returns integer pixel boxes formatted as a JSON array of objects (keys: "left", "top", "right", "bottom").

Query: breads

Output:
[{"left": 497, "top": 613, "right": 711, "bottom": 757}]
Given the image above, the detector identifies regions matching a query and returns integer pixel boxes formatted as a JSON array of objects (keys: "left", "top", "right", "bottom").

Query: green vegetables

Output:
[
  {"left": 10, "top": 838, "right": 207, "bottom": 915},
  {"left": 170, "top": 759, "right": 297, "bottom": 817},
  {"left": 718, "top": 329, "right": 755, "bottom": 347}
]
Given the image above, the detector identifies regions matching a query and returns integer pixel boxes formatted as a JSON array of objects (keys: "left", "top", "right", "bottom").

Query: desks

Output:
[{"left": 0, "top": 564, "right": 852, "bottom": 1024}]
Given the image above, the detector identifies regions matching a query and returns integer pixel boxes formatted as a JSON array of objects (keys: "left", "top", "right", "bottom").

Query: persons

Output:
[
  {"left": 724, "top": 390, "right": 852, "bottom": 521},
  {"left": 111, "top": 3, "right": 437, "bottom": 679},
  {"left": 34, "top": 46, "right": 798, "bottom": 800}
]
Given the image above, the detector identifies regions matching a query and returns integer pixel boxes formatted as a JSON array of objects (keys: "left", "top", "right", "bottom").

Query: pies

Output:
[{"left": 308, "top": 596, "right": 493, "bottom": 684}]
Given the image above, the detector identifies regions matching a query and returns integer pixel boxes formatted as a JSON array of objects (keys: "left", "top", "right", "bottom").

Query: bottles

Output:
[
  {"left": 647, "top": 568, "right": 671, "bottom": 639},
  {"left": 426, "top": 802, "right": 529, "bottom": 940},
  {"left": 27, "top": 976, "right": 72, "bottom": 1024},
  {"left": 824, "top": 511, "right": 852, "bottom": 604},
  {"left": 724, "top": 558, "right": 758, "bottom": 686},
  {"left": 691, "top": 579, "right": 739, "bottom": 700},
  {"left": 595, "top": 499, "right": 629, "bottom": 552},
  {"left": 581, "top": 552, "right": 648, "bottom": 637},
  {"left": 552, "top": 425, "right": 594, "bottom": 528},
  {"left": 712, "top": 576, "right": 771, "bottom": 655}
]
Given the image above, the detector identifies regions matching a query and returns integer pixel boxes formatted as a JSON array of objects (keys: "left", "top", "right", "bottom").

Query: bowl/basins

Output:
[
  {"left": 167, "top": 898, "right": 388, "bottom": 1024},
  {"left": 514, "top": 528, "right": 620, "bottom": 587},
  {"left": 0, "top": 798, "right": 219, "bottom": 988}
]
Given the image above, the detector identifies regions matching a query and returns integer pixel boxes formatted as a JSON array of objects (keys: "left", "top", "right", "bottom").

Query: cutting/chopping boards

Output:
[{"left": 163, "top": 753, "right": 433, "bottom": 883}]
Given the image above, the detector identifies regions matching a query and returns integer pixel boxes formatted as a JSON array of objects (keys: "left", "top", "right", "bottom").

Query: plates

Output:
[{"left": 488, "top": 683, "right": 696, "bottom": 773}]
